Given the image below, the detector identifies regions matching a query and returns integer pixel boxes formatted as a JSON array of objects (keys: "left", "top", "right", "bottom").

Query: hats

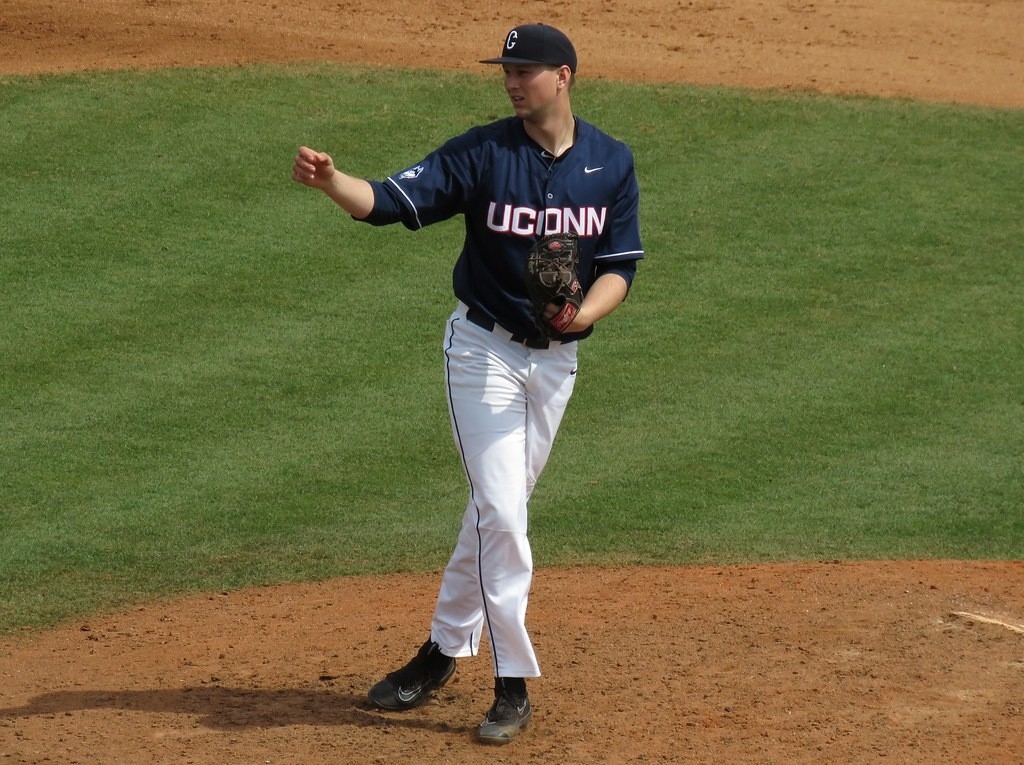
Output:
[{"left": 479, "top": 23, "right": 578, "bottom": 73}]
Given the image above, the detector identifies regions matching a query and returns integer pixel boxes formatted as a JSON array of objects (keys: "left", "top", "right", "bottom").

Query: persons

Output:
[{"left": 289, "top": 20, "right": 645, "bottom": 747}]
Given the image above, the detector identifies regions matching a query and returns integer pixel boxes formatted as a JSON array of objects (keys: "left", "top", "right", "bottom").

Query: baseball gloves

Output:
[{"left": 529, "top": 230, "right": 585, "bottom": 335}]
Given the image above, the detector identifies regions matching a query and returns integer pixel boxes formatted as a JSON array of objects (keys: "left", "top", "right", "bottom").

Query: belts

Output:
[{"left": 466, "top": 308, "right": 573, "bottom": 350}]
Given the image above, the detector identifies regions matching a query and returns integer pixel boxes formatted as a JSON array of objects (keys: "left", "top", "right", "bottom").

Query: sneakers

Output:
[
  {"left": 368, "top": 636, "right": 456, "bottom": 711},
  {"left": 478, "top": 691, "right": 530, "bottom": 743}
]
